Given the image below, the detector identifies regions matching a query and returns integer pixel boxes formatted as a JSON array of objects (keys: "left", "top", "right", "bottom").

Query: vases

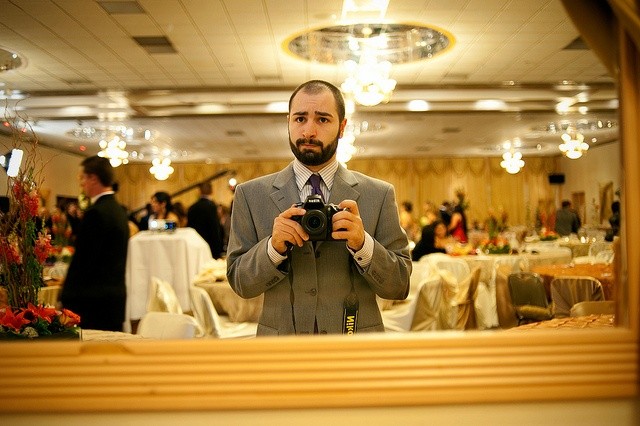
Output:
[{"left": 1, "top": 96, "right": 82, "bottom": 333}]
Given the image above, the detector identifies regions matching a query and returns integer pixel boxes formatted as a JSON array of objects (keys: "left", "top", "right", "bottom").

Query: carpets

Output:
[{"left": 309, "top": 174, "right": 325, "bottom": 201}]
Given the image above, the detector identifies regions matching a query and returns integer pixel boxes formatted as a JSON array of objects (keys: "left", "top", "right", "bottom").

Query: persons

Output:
[
  {"left": 398, "top": 191, "right": 470, "bottom": 261},
  {"left": 141, "top": 180, "right": 234, "bottom": 258},
  {"left": 554, "top": 200, "right": 582, "bottom": 235},
  {"left": 226, "top": 79, "right": 412, "bottom": 335},
  {"left": 0, "top": 157, "right": 139, "bottom": 332},
  {"left": 610, "top": 201, "right": 621, "bottom": 234}
]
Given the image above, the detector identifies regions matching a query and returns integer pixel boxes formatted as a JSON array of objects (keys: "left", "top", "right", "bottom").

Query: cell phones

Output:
[{"left": 291, "top": 195, "right": 346, "bottom": 242}]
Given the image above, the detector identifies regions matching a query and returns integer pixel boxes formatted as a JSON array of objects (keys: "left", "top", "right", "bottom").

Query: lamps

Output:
[
  {"left": 418, "top": 252, "right": 471, "bottom": 331},
  {"left": 150, "top": 276, "right": 174, "bottom": 313},
  {"left": 136, "top": 311, "right": 206, "bottom": 341},
  {"left": 187, "top": 286, "right": 259, "bottom": 338},
  {"left": 588, "top": 240, "right": 613, "bottom": 263},
  {"left": 380, "top": 277, "right": 460, "bottom": 332},
  {"left": 550, "top": 276, "right": 606, "bottom": 319},
  {"left": 569, "top": 300, "right": 615, "bottom": 317},
  {"left": 163, "top": 280, "right": 183, "bottom": 314},
  {"left": 508, "top": 272, "right": 553, "bottom": 326}
]
